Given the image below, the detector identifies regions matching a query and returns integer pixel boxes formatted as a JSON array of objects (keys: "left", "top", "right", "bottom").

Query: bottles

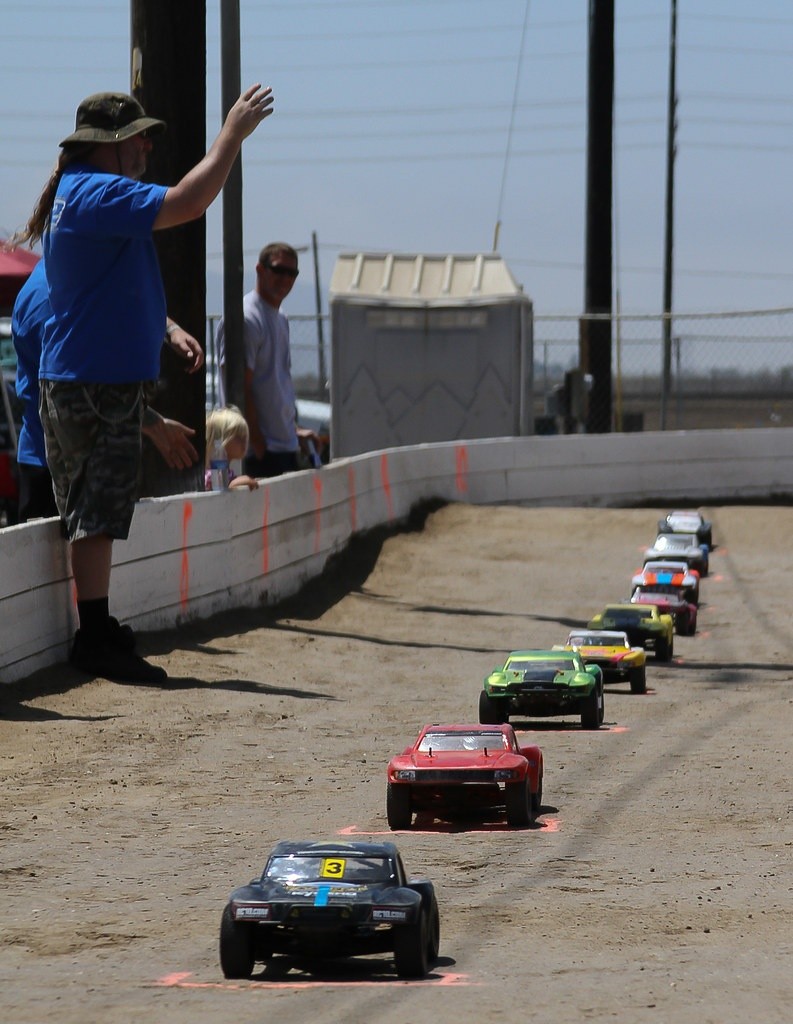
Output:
[{"left": 209, "top": 439, "right": 228, "bottom": 491}]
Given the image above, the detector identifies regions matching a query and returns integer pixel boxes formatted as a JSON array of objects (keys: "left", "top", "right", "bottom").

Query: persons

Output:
[
  {"left": 10, "top": 84, "right": 276, "bottom": 686},
  {"left": 214, "top": 243, "right": 322, "bottom": 479},
  {"left": 202, "top": 405, "right": 261, "bottom": 490}
]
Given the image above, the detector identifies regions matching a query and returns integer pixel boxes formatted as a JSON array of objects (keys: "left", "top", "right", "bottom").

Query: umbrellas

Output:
[{"left": 0, "top": 238, "right": 44, "bottom": 455}]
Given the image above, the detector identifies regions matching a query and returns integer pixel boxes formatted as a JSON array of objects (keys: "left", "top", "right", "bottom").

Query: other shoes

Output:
[{"left": 69, "top": 615, "right": 168, "bottom": 687}]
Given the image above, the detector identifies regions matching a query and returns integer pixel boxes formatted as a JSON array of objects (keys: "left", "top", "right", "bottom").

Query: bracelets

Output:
[{"left": 167, "top": 323, "right": 179, "bottom": 334}]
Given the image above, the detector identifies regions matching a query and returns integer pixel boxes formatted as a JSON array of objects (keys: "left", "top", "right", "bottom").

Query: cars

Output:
[
  {"left": 631, "top": 557, "right": 700, "bottom": 605},
  {"left": 629, "top": 585, "right": 699, "bottom": 637},
  {"left": 218, "top": 840, "right": 440, "bottom": 982},
  {"left": 551, "top": 628, "right": 648, "bottom": 695},
  {"left": 641, "top": 534, "right": 710, "bottom": 578},
  {"left": 655, "top": 510, "right": 713, "bottom": 552},
  {"left": 384, "top": 722, "right": 545, "bottom": 832},
  {"left": 585, "top": 602, "right": 677, "bottom": 663},
  {"left": 478, "top": 648, "right": 605, "bottom": 730}
]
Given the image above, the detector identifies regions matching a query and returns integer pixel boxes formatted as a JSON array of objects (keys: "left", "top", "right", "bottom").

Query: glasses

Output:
[
  {"left": 258, "top": 260, "right": 299, "bottom": 277},
  {"left": 134, "top": 128, "right": 148, "bottom": 138}
]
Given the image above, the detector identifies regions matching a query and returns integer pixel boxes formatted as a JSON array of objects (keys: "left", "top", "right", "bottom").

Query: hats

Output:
[{"left": 59, "top": 91, "right": 166, "bottom": 148}]
